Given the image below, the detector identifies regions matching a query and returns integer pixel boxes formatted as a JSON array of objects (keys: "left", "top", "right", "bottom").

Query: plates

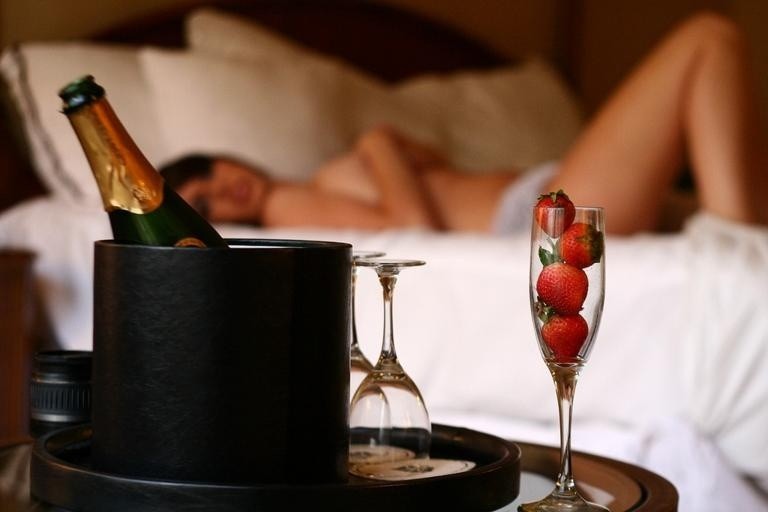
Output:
[{"left": 28, "top": 421, "right": 524, "bottom": 512}]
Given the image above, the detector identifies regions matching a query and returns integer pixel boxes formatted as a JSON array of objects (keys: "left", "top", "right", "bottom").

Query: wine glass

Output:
[
  {"left": 515, "top": 204, "right": 613, "bottom": 512},
  {"left": 350, "top": 250, "right": 432, "bottom": 478}
]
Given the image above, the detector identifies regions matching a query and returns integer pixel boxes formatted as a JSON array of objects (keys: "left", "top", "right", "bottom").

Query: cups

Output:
[
  {"left": 27, "top": 345, "right": 89, "bottom": 434},
  {"left": 87, "top": 233, "right": 349, "bottom": 481}
]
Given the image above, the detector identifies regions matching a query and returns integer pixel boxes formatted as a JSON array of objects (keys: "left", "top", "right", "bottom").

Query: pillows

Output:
[
  {"left": 4, "top": 42, "right": 188, "bottom": 212},
  {"left": 384, "top": 50, "right": 587, "bottom": 180},
  {"left": 191, "top": 10, "right": 446, "bottom": 174},
  {"left": 136, "top": 43, "right": 363, "bottom": 180}
]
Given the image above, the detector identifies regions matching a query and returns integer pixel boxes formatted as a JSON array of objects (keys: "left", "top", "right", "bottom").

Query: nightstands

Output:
[{"left": 0, "top": 247, "right": 41, "bottom": 450}]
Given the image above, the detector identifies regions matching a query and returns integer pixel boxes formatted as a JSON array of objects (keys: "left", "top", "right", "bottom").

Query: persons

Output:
[{"left": 146, "top": 8, "right": 767, "bottom": 240}]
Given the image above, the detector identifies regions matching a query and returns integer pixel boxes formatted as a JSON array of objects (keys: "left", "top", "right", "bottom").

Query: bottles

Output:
[{"left": 53, "top": 67, "right": 228, "bottom": 251}]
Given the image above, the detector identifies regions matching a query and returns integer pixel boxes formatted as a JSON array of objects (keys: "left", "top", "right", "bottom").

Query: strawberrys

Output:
[
  {"left": 541, "top": 312, "right": 588, "bottom": 358},
  {"left": 533, "top": 193, "right": 576, "bottom": 238},
  {"left": 536, "top": 262, "right": 588, "bottom": 315},
  {"left": 557, "top": 222, "right": 604, "bottom": 268}
]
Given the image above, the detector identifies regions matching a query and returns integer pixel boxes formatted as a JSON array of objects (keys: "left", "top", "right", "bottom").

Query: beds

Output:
[{"left": 0, "top": 2, "right": 768, "bottom": 512}]
